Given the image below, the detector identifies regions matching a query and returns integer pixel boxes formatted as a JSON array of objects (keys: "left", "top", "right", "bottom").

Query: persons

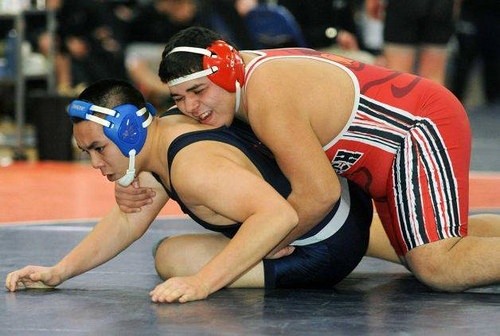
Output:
[
  {"left": 107, "top": 25, "right": 500, "bottom": 297},
  {"left": 0, "top": 69, "right": 498, "bottom": 304},
  {"left": 6, "top": 1, "right": 498, "bottom": 131}
]
